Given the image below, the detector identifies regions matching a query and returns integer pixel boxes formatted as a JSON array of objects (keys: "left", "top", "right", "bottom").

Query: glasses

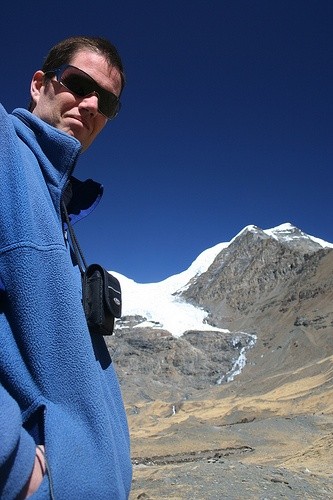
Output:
[{"left": 45, "top": 64, "right": 122, "bottom": 120}]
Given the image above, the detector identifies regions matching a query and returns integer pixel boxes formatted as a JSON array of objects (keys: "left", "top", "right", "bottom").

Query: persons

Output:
[{"left": 0, "top": 35, "right": 134, "bottom": 500}]
